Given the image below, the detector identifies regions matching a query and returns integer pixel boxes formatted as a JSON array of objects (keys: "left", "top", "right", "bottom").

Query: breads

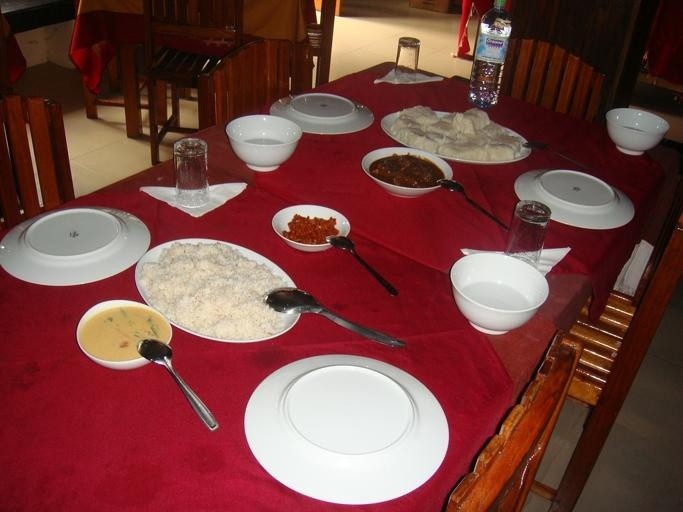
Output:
[{"left": 393, "top": 106, "right": 522, "bottom": 161}]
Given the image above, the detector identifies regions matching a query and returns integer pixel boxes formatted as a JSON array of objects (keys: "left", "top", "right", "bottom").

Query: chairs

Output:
[
  {"left": 75, "top": 1, "right": 149, "bottom": 118},
  {"left": 445, "top": 328, "right": 584, "bottom": 511},
  {"left": 142, "top": 0, "right": 243, "bottom": 165},
  {"left": 289, "top": 1, "right": 336, "bottom": 96},
  {"left": 499, "top": 38, "right": 605, "bottom": 122},
  {"left": 530, "top": 212, "right": 683, "bottom": 511},
  {"left": 197, "top": 39, "right": 292, "bottom": 130},
  {"left": 0, "top": 94, "right": 75, "bottom": 229}
]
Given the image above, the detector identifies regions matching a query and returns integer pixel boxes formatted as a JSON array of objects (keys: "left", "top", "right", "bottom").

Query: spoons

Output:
[
  {"left": 137, "top": 341, "right": 221, "bottom": 434},
  {"left": 437, "top": 177, "right": 511, "bottom": 233},
  {"left": 325, "top": 234, "right": 399, "bottom": 298},
  {"left": 520, "top": 139, "right": 583, "bottom": 170},
  {"left": 262, "top": 287, "right": 409, "bottom": 350}
]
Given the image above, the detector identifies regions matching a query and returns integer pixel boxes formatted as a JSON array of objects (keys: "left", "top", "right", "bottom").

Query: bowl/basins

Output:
[
  {"left": 224, "top": 114, "right": 301, "bottom": 174},
  {"left": 449, "top": 253, "right": 550, "bottom": 338},
  {"left": 604, "top": 105, "right": 670, "bottom": 158}
]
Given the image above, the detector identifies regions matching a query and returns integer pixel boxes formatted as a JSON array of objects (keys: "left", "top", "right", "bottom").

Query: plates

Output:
[
  {"left": 0, "top": 204, "right": 151, "bottom": 285},
  {"left": 270, "top": 92, "right": 374, "bottom": 136},
  {"left": 512, "top": 167, "right": 637, "bottom": 231},
  {"left": 362, "top": 147, "right": 453, "bottom": 200},
  {"left": 380, "top": 108, "right": 534, "bottom": 164},
  {"left": 134, "top": 237, "right": 301, "bottom": 342},
  {"left": 242, "top": 352, "right": 453, "bottom": 506},
  {"left": 272, "top": 203, "right": 352, "bottom": 255},
  {"left": 73, "top": 298, "right": 175, "bottom": 374}
]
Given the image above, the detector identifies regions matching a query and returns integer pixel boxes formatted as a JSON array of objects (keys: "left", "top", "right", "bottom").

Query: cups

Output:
[
  {"left": 394, "top": 35, "right": 420, "bottom": 82},
  {"left": 505, "top": 198, "right": 551, "bottom": 268},
  {"left": 171, "top": 138, "right": 209, "bottom": 208}
]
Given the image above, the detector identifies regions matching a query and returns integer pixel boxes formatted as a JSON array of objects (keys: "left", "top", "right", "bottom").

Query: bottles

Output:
[{"left": 467, "top": 0, "right": 512, "bottom": 106}]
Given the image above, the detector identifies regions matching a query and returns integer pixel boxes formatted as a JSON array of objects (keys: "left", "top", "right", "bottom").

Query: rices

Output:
[{"left": 142, "top": 240, "right": 286, "bottom": 336}]
